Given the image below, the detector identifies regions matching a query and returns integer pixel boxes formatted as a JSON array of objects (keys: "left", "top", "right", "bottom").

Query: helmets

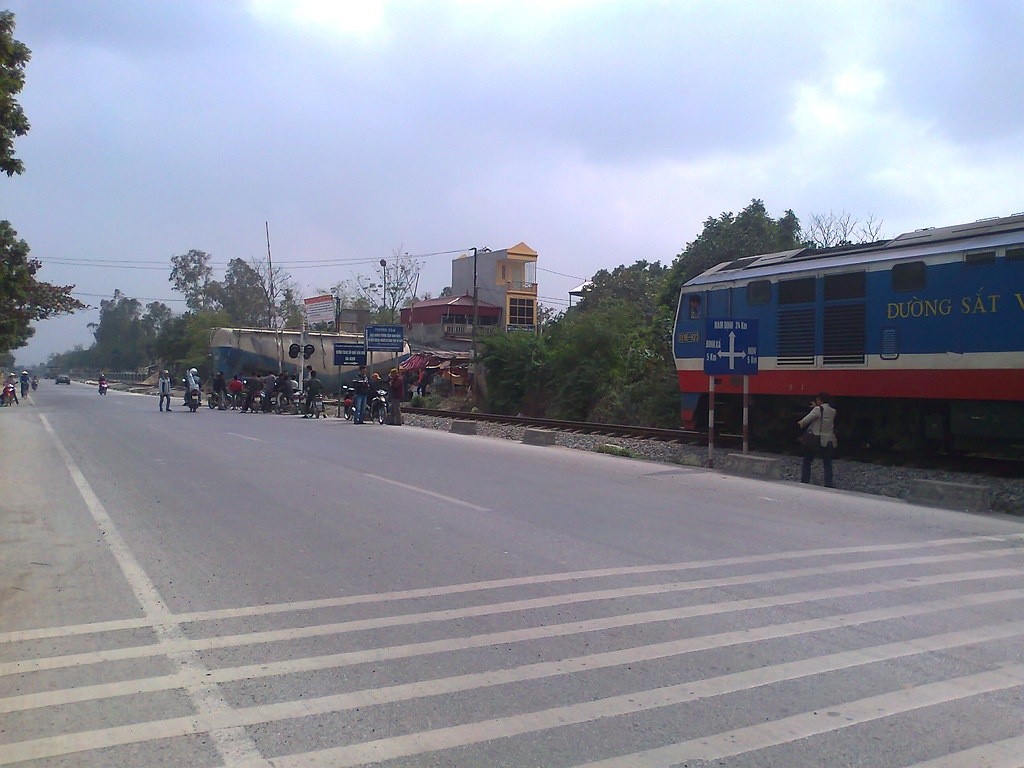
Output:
[
  {"left": 191, "top": 368, "right": 197, "bottom": 375},
  {"left": 101, "top": 374, "right": 104, "bottom": 377}
]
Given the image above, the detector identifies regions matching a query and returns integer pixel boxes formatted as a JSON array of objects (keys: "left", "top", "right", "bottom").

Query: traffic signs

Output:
[
  {"left": 703, "top": 315, "right": 759, "bottom": 374},
  {"left": 333, "top": 343, "right": 367, "bottom": 366}
]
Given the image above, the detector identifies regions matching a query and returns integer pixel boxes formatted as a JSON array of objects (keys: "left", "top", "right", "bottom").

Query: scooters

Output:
[{"left": 99, "top": 379, "right": 107, "bottom": 395}]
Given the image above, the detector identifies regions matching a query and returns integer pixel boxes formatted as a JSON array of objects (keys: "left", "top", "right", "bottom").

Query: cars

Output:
[{"left": 55, "top": 373, "right": 70, "bottom": 385}]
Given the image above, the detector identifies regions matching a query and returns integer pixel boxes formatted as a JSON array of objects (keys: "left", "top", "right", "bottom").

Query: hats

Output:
[
  {"left": 9, "top": 373, "right": 15, "bottom": 376},
  {"left": 22, "top": 371, "right": 28, "bottom": 374},
  {"left": 164, "top": 370, "right": 169, "bottom": 374},
  {"left": 373, "top": 373, "right": 381, "bottom": 379}
]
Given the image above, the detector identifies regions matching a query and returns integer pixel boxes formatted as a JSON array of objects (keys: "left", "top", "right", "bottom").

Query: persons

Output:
[
  {"left": 213, "top": 365, "right": 328, "bottom": 418},
  {"left": 31, "top": 375, "right": 39, "bottom": 389},
  {"left": 99, "top": 373, "right": 108, "bottom": 392},
  {"left": 20, "top": 370, "right": 30, "bottom": 397},
  {"left": 414, "top": 367, "right": 430, "bottom": 396},
  {"left": 183, "top": 368, "right": 203, "bottom": 405},
  {"left": 3, "top": 373, "right": 19, "bottom": 404},
  {"left": 797, "top": 391, "right": 837, "bottom": 488},
  {"left": 158, "top": 369, "right": 172, "bottom": 411},
  {"left": 353, "top": 365, "right": 403, "bottom": 426}
]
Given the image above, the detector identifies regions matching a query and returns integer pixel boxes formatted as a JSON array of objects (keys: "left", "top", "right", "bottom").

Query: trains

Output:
[{"left": 673, "top": 211, "right": 1024, "bottom": 472}]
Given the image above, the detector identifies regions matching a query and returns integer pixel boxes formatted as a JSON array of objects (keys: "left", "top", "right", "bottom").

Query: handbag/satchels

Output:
[{"left": 801, "top": 430, "right": 820, "bottom": 453}]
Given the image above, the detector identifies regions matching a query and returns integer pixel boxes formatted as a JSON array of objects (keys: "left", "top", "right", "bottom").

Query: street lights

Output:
[
  {"left": 469, "top": 246, "right": 477, "bottom": 364},
  {"left": 379, "top": 258, "right": 386, "bottom": 325}
]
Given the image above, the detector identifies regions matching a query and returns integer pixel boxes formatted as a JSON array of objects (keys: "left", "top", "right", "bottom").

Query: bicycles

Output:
[{"left": 22, "top": 380, "right": 30, "bottom": 400}]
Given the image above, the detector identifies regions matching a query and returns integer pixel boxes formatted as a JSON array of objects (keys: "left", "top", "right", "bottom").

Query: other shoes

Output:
[
  {"left": 167, "top": 409, "right": 172, "bottom": 411},
  {"left": 160, "top": 409, "right": 162, "bottom": 411},
  {"left": 323, "top": 414, "right": 327, "bottom": 417},
  {"left": 301, "top": 416, "right": 307, "bottom": 418}
]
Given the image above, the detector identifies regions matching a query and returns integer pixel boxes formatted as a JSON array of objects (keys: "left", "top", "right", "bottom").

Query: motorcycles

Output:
[
  {"left": 369, "top": 389, "right": 389, "bottom": 425},
  {"left": 208, "top": 380, "right": 324, "bottom": 419},
  {"left": 342, "top": 385, "right": 357, "bottom": 420},
  {"left": 3, "top": 381, "right": 18, "bottom": 406},
  {"left": 32, "top": 379, "right": 38, "bottom": 391},
  {"left": 182, "top": 378, "right": 202, "bottom": 412}
]
